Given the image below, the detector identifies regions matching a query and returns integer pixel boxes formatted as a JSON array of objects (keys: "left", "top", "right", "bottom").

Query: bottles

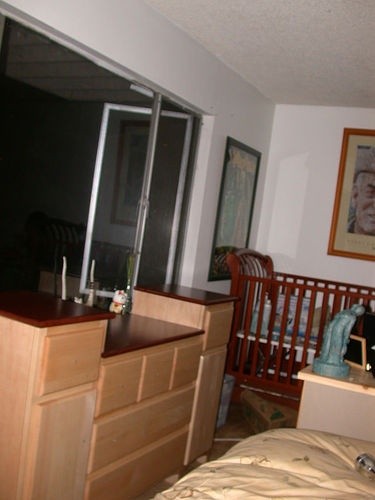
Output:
[
  {"left": 86, "top": 282, "right": 97, "bottom": 307},
  {"left": 260, "top": 291, "right": 272, "bottom": 335},
  {"left": 354, "top": 452, "right": 375, "bottom": 484}
]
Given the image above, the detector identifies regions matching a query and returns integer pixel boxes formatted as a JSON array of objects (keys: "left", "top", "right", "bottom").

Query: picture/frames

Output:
[
  {"left": 326, "top": 128, "right": 375, "bottom": 262},
  {"left": 110, "top": 119, "right": 167, "bottom": 228},
  {"left": 207, "top": 135, "right": 262, "bottom": 284}
]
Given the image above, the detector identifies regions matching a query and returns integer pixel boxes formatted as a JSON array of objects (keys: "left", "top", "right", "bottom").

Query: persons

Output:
[{"left": 319, "top": 304, "right": 366, "bottom": 365}]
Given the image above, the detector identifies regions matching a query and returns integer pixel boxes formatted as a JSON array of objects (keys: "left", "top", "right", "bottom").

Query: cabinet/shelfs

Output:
[
  {"left": 292, "top": 361, "right": 374, "bottom": 441},
  {"left": 72, "top": 312, "right": 206, "bottom": 500},
  {"left": 0, "top": 291, "right": 115, "bottom": 500},
  {"left": 37, "top": 265, "right": 115, "bottom": 310},
  {"left": 129, "top": 283, "right": 237, "bottom": 465}
]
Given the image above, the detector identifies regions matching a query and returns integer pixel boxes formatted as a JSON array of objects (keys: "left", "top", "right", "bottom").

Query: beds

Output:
[
  {"left": 224, "top": 249, "right": 374, "bottom": 411},
  {"left": 51, "top": 219, "right": 135, "bottom": 284},
  {"left": 148, "top": 428, "right": 374, "bottom": 500}
]
